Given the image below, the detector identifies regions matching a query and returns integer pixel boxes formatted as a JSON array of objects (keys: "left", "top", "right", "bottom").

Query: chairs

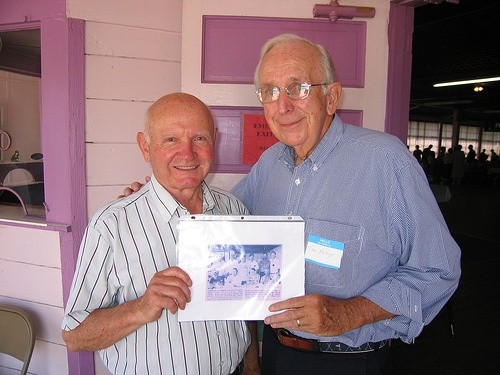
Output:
[{"left": 0, "top": 305, "right": 36, "bottom": 375}]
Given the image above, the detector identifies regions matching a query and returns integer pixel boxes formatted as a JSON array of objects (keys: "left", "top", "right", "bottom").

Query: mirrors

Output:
[{"left": 0, "top": 28, "right": 47, "bottom": 225}]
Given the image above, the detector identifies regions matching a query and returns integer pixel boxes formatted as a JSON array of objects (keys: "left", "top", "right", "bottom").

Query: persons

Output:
[
  {"left": 117, "top": 34, "right": 462, "bottom": 375},
  {"left": 61, "top": 92, "right": 261, "bottom": 375},
  {"left": 407, "top": 144, "right": 500, "bottom": 186}
]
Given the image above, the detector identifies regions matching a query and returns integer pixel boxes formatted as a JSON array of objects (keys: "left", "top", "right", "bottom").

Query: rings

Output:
[{"left": 296, "top": 319, "right": 301, "bottom": 326}]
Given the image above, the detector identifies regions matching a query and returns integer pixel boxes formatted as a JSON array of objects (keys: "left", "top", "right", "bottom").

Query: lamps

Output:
[{"left": 433, "top": 75, "right": 500, "bottom": 87}]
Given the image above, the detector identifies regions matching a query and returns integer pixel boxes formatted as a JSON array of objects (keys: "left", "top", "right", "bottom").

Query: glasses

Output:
[{"left": 255, "top": 81, "right": 328, "bottom": 104}]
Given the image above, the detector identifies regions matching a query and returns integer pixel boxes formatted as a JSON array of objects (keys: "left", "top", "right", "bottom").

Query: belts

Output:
[{"left": 275, "top": 328, "right": 391, "bottom": 353}]
